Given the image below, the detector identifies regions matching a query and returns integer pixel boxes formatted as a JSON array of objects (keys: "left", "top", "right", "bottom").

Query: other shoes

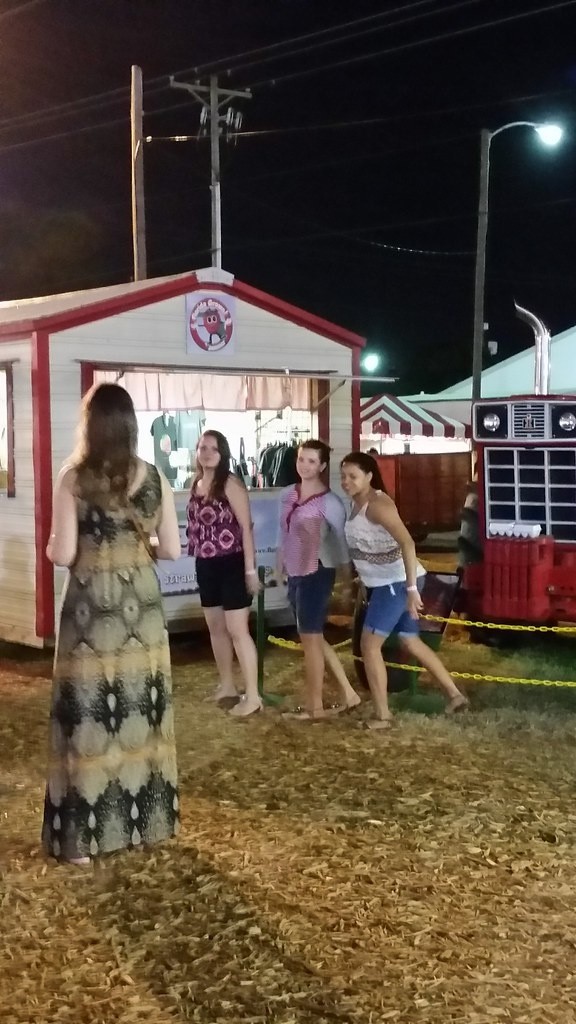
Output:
[
  {"left": 204, "top": 691, "right": 237, "bottom": 702},
  {"left": 65, "top": 856, "right": 90, "bottom": 864},
  {"left": 227, "top": 694, "right": 262, "bottom": 717}
]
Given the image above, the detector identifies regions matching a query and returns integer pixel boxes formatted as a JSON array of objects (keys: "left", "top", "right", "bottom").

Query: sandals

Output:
[
  {"left": 355, "top": 714, "right": 396, "bottom": 732},
  {"left": 281, "top": 705, "right": 325, "bottom": 722},
  {"left": 324, "top": 695, "right": 361, "bottom": 716},
  {"left": 442, "top": 694, "right": 469, "bottom": 716}
]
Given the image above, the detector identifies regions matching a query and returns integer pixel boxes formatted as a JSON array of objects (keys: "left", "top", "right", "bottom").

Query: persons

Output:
[
  {"left": 185, "top": 429, "right": 266, "bottom": 718},
  {"left": 44, "top": 382, "right": 181, "bottom": 861},
  {"left": 245, "top": 457, "right": 269, "bottom": 491},
  {"left": 276, "top": 440, "right": 362, "bottom": 719},
  {"left": 339, "top": 451, "right": 473, "bottom": 730}
]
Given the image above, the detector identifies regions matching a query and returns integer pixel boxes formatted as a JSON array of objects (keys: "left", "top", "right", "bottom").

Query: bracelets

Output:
[
  {"left": 243, "top": 567, "right": 254, "bottom": 575},
  {"left": 405, "top": 583, "right": 419, "bottom": 588}
]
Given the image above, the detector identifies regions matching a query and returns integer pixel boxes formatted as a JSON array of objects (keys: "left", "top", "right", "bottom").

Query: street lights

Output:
[{"left": 468, "top": 115, "right": 566, "bottom": 621}]
